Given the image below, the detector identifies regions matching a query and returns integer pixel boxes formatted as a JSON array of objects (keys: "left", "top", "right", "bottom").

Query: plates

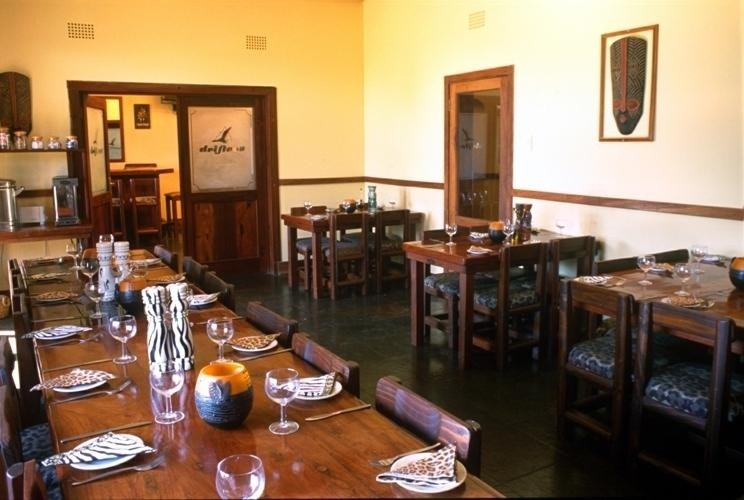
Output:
[
  {"left": 650, "top": 267, "right": 666, "bottom": 275},
  {"left": 36, "top": 291, "right": 70, "bottom": 302},
  {"left": 391, "top": 451, "right": 466, "bottom": 494},
  {"left": 70, "top": 434, "right": 144, "bottom": 471},
  {"left": 35, "top": 327, "right": 77, "bottom": 341},
  {"left": 282, "top": 377, "right": 343, "bottom": 401},
  {"left": 231, "top": 335, "right": 278, "bottom": 352},
  {"left": 665, "top": 290, "right": 716, "bottom": 312},
  {"left": 157, "top": 274, "right": 185, "bottom": 284},
  {"left": 187, "top": 294, "right": 217, "bottom": 309},
  {"left": 34, "top": 273, "right": 62, "bottom": 282},
  {"left": 468, "top": 247, "right": 489, "bottom": 255},
  {"left": 53, "top": 370, "right": 108, "bottom": 393},
  {"left": 573, "top": 273, "right": 626, "bottom": 287}
]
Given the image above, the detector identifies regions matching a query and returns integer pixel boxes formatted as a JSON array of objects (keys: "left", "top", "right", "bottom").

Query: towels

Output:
[
  {"left": 636, "top": 262, "right": 674, "bottom": 270},
  {"left": 471, "top": 232, "right": 487, "bottom": 238},
  {"left": 178, "top": 292, "right": 221, "bottom": 302},
  {"left": 31, "top": 369, "right": 121, "bottom": 391},
  {"left": 41, "top": 431, "right": 159, "bottom": 467},
  {"left": 222, "top": 333, "right": 284, "bottom": 349},
  {"left": 666, "top": 296, "right": 708, "bottom": 307},
  {"left": 27, "top": 255, "right": 59, "bottom": 263},
  {"left": 282, "top": 372, "right": 338, "bottom": 397},
  {"left": 22, "top": 324, "right": 93, "bottom": 338},
  {"left": 374, "top": 441, "right": 457, "bottom": 487},
  {"left": 572, "top": 276, "right": 612, "bottom": 284},
  {"left": 468, "top": 245, "right": 493, "bottom": 252},
  {"left": 147, "top": 273, "right": 188, "bottom": 282},
  {"left": 697, "top": 256, "right": 728, "bottom": 261},
  {"left": 24, "top": 272, "right": 72, "bottom": 279},
  {"left": 129, "top": 257, "right": 164, "bottom": 267},
  {"left": 24, "top": 291, "right": 80, "bottom": 299}
]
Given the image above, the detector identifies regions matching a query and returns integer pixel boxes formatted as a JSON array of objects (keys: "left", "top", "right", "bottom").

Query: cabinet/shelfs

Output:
[{"left": 0, "top": 147, "right": 93, "bottom": 293}]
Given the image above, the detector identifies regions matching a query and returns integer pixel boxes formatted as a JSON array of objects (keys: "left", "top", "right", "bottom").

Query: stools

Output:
[{"left": 166, "top": 192, "right": 181, "bottom": 244}]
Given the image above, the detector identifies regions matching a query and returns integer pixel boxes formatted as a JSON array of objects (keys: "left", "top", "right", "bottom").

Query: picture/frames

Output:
[
  {"left": 598, "top": 23, "right": 660, "bottom": 140},
  {"left": 135, "top": 103, "right": 150, "bottom": 130}
]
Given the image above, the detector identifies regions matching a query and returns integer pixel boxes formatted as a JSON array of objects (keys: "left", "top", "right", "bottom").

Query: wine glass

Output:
[
  {"left": 691, "top": 245, "right": 706, "bottom": 270},
  {"left": 264, "top": 368, "right": 300, "bottom": 435},
  {"left": 150, "top": 362, "right": 185, "bottom": 424},
  {"left": 82, "top": 256, "right": 99, "bottom": 282},
  {"left": 446, "top": 221, "right": 457, "bottom": 246},
  {"left": 207, "top": 316, "right": 234, "bottom": 363},
  {"left": 66, "top": 241, "right": 83, "bottom": 271},
  {"left": 304, "top": 200, "right": 312, "bottom": 216},
  {"left": 637, "top": 256, "right": 655, "bottom": 286},
  {"left": 99, "top": 233, "right": 114, "bottom": 243},
  {"left": 557, "top": 218, "right": 565, "bottom": 235},
  {"left": 503, "top": 224, "right": 513, "bottom": 244},
  {"left": 216, "top": 454, "right": 265, "bottom": 500},
  {"left": 674, "top": 263, "right": 692, "bottom": 296},
  {"left": 109, "top": 314, "right": 138, "bottom": 364},
  {"left": 87, "top": 281, "right": 108, "bottom": 319}
]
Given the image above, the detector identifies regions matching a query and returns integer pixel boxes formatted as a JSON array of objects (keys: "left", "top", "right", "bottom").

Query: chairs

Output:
[
  {"left": 339, "top": 210, "right": 410, "bottom": 296},
  {"left": 178, "top": 254, "right": 208, "bottom": 287},
  {"left": 200, "top": 271, "right": 236, "bottom": 305},
  {"left": 154, "top": 244, "right": 176, "bottom": 267},
  {"left": 12, "top": 293, "right": 35, "bottom": 389},
  {"left": 291, "top": 331, "right": 359, "bottom": 395},
  {"left": 292, "top": 206, "right": 340, "bottom": 291},
  {"left": 626, "top": 298, "right": 744, "bottom": 500},
  {"left": 109, "top": 178, "right": 126, "bottom": 242},
  {"left": 7, "top": 458, "right": 64, "bottom": 499},
  {"left": 245, "top": 301, "right": 296, "bottom": 348},
  {"left": 555, "top": 277, "right": 669, "bottom": 476},
  {"left": 340, "top": 204, "right": 376, "bottom": 277},
  {"left": 321, "top": 212, "right": 370, "bottom": 301},
  {"left": 423, "top": 230, "right": 498, "bottom": 350},
  {"left": 1, "top": 384, "right": 55, "bottom": 462},
  {"left": 514, "top": 235, "right": 599, "bottom": 361},
  {"left": 8, "top": 258, "right": 21, "bottom": 275},
  {"left": 466, "top": 224, "right": 530, "bottom": 328},
  {"left": 1, "top": 336, "right": 41, "bottom": 397},
  {"left": 650, "top": 247, "right": 691, "bottom": 268},
  {"left": 456, "top": 242, "right": 546, "bottom": 372},
  {"left": 128, "top": 178, "right": 163, "bottom": 243},
  {"left": 589, "top": 252, "right": 689, "bottom": 365},
  {"left": 370, "top": 376, "right": 483, "bottom": 475},
  {"left": 9, "top": 274, "right": 29, "bottom": 296}
]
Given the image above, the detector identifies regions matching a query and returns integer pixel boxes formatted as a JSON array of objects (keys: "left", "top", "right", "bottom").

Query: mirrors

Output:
[{"left": 104, "top": 95, "right": 126, "bottom": 164}]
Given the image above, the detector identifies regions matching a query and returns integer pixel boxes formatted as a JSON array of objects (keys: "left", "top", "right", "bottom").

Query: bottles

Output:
[
  {"left": 0, "top": 127, "right": 10, "bottom": 152},
  {"left": 48, "top": 137, "right": 61, "bottom": 149},
  {"left": 15, "top": 131, "right": 28, "bottom": 150},
  {"left": 31, "top": 136, "right": 44, "bottom": 149},
  {"left": 65, "top": 136, "right": 78, "bottom": 148}
]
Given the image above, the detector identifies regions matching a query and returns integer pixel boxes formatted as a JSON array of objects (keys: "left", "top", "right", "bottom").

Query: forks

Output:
[
  {"left": 367, "top": 443, "right": 442, "bottom": 467},
  {"left": 50, "top": 378, "right": 132, "bottom": 405},
  {"left": 71, "top": 455, "right": 166, "bottom": 486}
]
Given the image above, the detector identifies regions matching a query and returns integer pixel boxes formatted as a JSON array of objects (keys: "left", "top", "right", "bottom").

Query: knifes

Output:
[
  {"left": 236, "top": 347, "right": 294, "bottom": 362},
  {"left": 30, "top": 315, "right": 81, "bottom": 323},
  {"left": 304, "top": 404, "right": 372, "bottom": 422},
  {"left": 42, "top": 358, "right": 113, "bottom": 375},
  {"left": 195, "top": 316, "right": 244, "bottom": 324},
  {"left": 60, "top": 420, "right": 153, "bottom": 443}
]
